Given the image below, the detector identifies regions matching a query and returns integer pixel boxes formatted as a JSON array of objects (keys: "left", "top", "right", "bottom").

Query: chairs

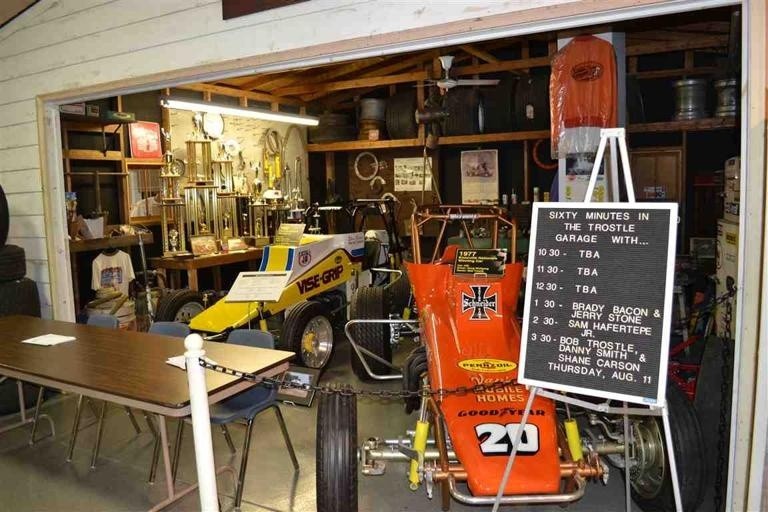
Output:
[
  {"left": 165, "top": 326, "right": 302, "bottom": 512},
  {"left": 24, "top": 311, "right": 143, "bottom": 466},
  {"left": 87, "top": 316, "right": 195, "bottom": 486}
]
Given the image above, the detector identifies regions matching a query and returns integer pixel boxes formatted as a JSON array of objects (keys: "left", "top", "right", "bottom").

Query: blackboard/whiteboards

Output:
[{"left": 517, "top": 202, "right": 678, "bottom": 405}]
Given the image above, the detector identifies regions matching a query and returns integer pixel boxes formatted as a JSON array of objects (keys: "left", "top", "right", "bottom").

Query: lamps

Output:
[{"left": 154, "top": 85, "right": 321, "bottom": 128}]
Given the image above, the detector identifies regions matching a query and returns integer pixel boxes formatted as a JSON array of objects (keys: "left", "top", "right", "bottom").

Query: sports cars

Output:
[
  {"left": 315, "top": 205, "right": 709, "bottom": 511},
  {"left": 157, "top": 200, "right": 392, "bottom": 375}
]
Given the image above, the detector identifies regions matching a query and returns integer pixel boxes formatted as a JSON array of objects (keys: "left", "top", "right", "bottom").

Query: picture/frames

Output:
[
  {"left": 274, "top": 362, "right": 324, "bottom": 408},
  {"left": 199, "top": 107, "right": 225, "bottom": 139},
  {"left": 189, "top": 233, "right": 221, "bottom": 257}
]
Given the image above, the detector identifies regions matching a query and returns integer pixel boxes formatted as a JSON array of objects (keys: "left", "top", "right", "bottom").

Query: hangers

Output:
[{"left": 99, "top": 235, "right": 118, "bottom": 254}]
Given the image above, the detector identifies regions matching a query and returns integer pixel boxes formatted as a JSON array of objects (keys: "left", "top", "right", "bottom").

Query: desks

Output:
[
  {"left": 0, "top": 310, "right": 296, "bottom": 512},
  {"left": 147, "top": 241, "right": 268, "bottom": 298}
]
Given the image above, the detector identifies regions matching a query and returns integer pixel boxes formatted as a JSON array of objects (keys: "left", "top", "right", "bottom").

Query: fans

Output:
[{"left": 405, "top": 45, "right": 506, "bottom": 93}]
